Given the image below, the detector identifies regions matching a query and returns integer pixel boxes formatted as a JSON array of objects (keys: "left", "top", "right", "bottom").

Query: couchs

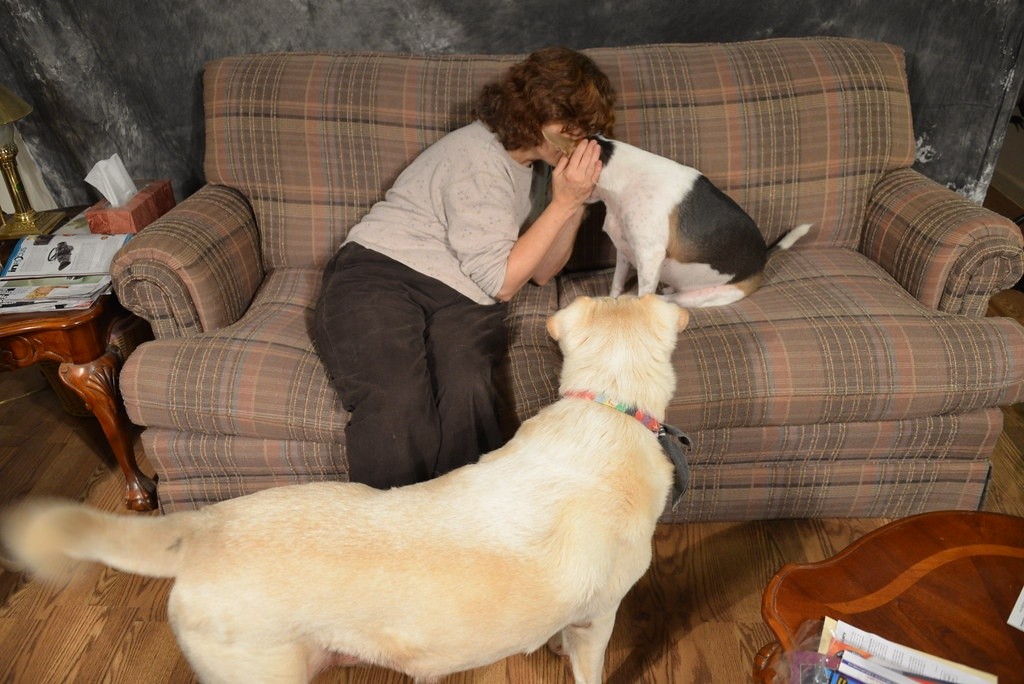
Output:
[{"left": 108, "top": 32, "right": 1024, "bottom": 525}]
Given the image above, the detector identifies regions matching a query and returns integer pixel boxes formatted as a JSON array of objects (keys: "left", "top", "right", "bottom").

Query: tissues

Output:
[{"left": 83, "top": 152, "right": 176, "bottom": 235}]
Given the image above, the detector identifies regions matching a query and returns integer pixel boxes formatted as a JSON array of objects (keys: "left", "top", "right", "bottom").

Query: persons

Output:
[{"left": 311, "top": 47, "right": 616, "bottom": 491}]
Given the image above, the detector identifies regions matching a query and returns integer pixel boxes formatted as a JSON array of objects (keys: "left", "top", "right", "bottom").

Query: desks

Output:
[
  {"left": 0, "top": 205, "right": 162, "bottom": 514},
  {"left": 751, "top": 511, "right": 1024, "bottom": 684}
]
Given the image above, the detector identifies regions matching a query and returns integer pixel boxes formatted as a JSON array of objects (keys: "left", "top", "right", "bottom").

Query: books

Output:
[{"left": 1, "top": 233, "right": 133, "bottom": 314}]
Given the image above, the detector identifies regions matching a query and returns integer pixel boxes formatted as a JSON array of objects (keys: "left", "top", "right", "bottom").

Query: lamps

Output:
[{"left": 0, "top": 82, "right": 68, "bottom": 241}]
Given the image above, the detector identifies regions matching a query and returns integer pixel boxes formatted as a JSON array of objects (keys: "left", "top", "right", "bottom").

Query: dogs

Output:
[
  {"left": 0, "top": 293, "right": 691, "bottom": 684},
  {"left": 541, "top": 126, "right": 814, "bottom": 312}
]
